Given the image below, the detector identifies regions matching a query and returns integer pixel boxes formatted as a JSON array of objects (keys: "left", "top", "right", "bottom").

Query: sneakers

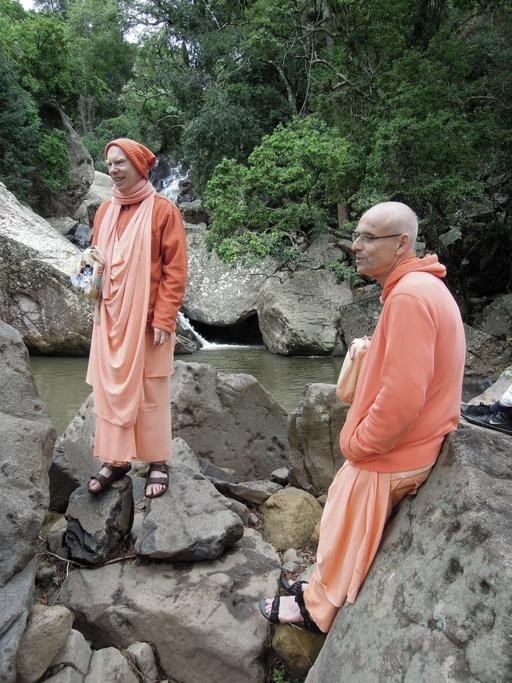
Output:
[{"left": 459, "top": 400, "right": 512, "bottom": 436}]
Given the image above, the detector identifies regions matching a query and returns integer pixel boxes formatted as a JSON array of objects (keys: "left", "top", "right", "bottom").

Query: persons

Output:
[
  {"left": 76, "top": 137, "right": 190, "bottom": 497},
  {"left": 260, "top": 198, "right": 470, "bottom": 635}
]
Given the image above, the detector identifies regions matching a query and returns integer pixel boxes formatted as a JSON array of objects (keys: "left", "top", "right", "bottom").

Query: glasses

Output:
[{"left": 350, "top": 231, "right": 402, "bottom": 244}]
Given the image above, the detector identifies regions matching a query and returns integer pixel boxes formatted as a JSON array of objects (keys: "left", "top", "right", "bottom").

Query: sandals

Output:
[
  {"left": 88, "top": 462, "right": 132, "bottom": 494},
  {"left": 259, "top": 577, "right": 317, "bottom": 629},
  {"left": 144, "top": 463, "right": 169, "bottom": 498}
]
